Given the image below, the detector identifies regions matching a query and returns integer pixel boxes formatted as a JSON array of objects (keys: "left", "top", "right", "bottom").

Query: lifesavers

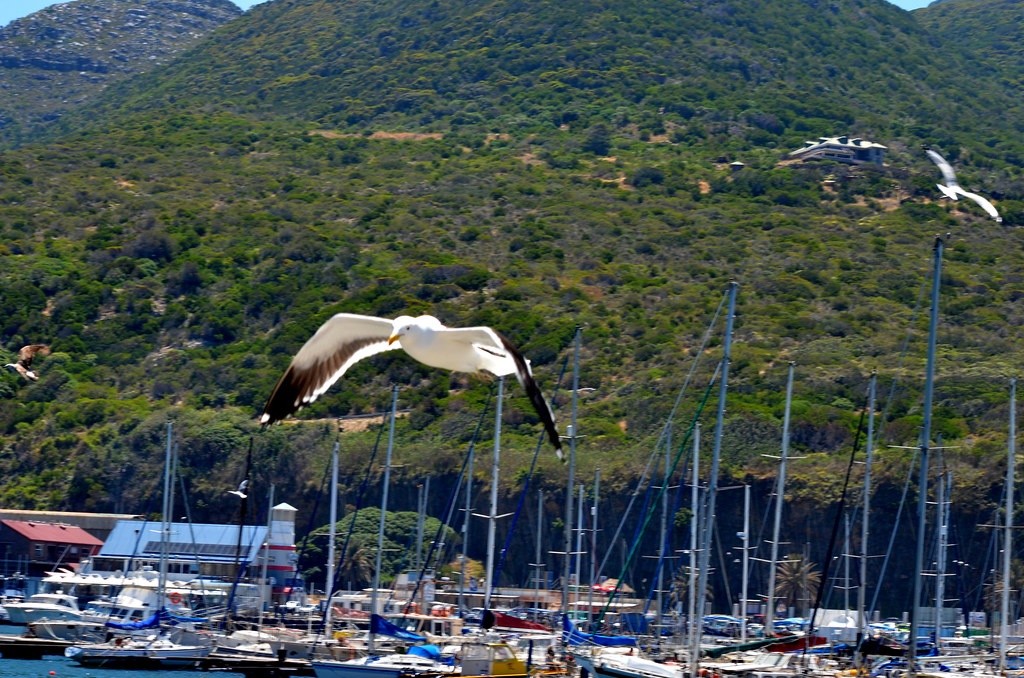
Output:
[{"left": 170, "top": 592, "right": 182, "bottom": 604}]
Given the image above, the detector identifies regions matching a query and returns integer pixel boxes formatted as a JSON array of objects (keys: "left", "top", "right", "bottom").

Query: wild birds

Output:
[
  {"left": 921, "top": 146, "right": 1004, "bottom": 225},
  {"left": 3, "top": 344, "right": 51, "bottom": 382},
  {"left": 255, "top": 313, "right": 569, "bottom": 468}
]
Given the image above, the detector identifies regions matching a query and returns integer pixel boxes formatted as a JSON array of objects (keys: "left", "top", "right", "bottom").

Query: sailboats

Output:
[{"left": 0, "top": 232, "right": 1024, "bottom": 678}]
